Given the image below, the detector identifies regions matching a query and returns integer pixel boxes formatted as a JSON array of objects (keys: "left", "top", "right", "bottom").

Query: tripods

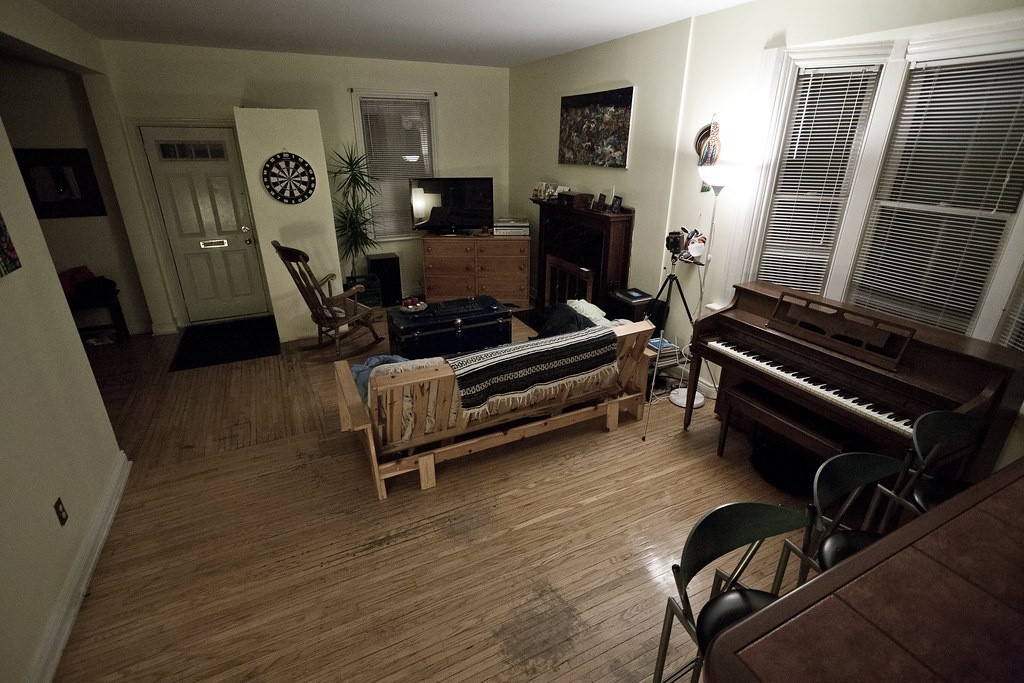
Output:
[{"left": 643, "top": 255, "right": 719, "bottom": 442}]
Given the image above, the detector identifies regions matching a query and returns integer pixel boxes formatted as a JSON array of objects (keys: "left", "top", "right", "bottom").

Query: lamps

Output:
[{"left": 669, "top": 163, "right": 729, "bottom": 407}]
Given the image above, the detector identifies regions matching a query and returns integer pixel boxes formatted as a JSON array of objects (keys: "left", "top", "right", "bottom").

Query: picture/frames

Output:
[{"left": 13, "top": 148, "right": 108, "bottom": 219}]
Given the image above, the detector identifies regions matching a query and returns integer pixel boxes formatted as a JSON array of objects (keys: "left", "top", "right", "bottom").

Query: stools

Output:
[{"left": 68, "top": 290, "right": 130, "bottom": 345}]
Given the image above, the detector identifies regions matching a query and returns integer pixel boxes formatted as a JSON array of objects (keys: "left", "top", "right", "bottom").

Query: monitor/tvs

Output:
[{"left": 409, "top": 177, "right": 494, "bottom": 236}]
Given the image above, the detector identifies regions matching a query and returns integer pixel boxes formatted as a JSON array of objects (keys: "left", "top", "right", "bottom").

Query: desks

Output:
[
  {"left": 708, "top": 459, "right": 1024, "bottom": 683},
  {"left": 607, "top": 290, "right": 670, "bottom": 341}
]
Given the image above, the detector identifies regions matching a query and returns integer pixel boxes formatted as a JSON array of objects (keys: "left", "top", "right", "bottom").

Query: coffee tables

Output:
[{"left": 385, "top": 297, "right": 512, "bottom": 355}]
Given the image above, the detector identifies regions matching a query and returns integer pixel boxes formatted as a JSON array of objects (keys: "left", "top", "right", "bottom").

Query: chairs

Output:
[
  {"left": 270, "top": 241, "right": 381, "bottom": 364},
  {"left": 653, "top": 387, "right": 1001, "bottom": 683}
]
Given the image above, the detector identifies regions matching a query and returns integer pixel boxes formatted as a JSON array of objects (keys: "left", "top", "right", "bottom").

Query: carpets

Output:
[{"left": 169, "top": 313, "right": 282, "bottom": 372}]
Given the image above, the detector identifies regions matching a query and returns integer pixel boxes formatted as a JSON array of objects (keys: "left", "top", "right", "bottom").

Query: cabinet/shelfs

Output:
[
  {"left": 537, "top": 199, "right": 635, "bottom": 314},
  {"left": 421, "top": 236, "right": 531, "bottom": 309}
]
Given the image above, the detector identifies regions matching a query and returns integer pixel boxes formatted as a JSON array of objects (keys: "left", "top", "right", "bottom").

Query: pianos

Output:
[{"left": 682, "top": 278, "right": 1024, "bottom": 532}]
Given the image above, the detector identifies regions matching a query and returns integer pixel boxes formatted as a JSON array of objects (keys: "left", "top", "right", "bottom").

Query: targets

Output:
[{"left": 262, "top": 152, "right": 317, "bottom": 204}]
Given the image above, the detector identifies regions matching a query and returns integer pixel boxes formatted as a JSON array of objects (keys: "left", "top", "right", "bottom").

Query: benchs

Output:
[
  {"left": 712, "top": 388, "right": 849, "bottom": 465},
  {"left": 332, "top": 318, "right": 657, "bottom": 499}
]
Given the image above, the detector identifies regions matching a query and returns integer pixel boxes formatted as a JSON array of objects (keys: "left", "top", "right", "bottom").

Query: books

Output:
[{"left": 647, "top": 337, "right": 680, "bottom": 367}]
